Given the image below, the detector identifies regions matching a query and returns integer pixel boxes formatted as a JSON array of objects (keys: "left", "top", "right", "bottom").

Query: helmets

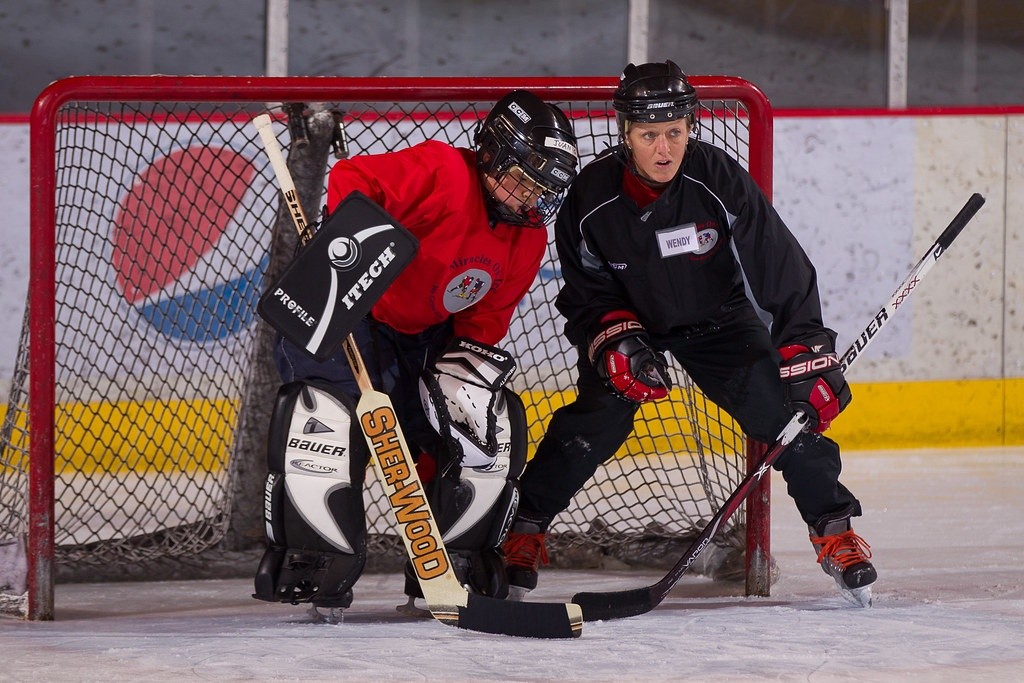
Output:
[
  {"left": 473, "top": 90, "right": 577, "bottom": 195},
  {"left": 613, "top": 59, "right": 698, "bottom": 122}
]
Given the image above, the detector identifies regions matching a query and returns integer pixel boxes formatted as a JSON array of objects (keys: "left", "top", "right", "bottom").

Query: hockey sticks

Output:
[
  {"left": 254, "top": 113, "right": 586, "bottom": 640},
  {"left": 571, "top": 191, "right": 984, "bottom": 623}
]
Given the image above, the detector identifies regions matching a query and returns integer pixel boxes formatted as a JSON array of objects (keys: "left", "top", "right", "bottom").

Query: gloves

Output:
[
  {"left": 782, "top": 334, "right": 851, "bottom": 434},
  {"left": 587, "top": 311, "right": 672, "bottom": 404}
]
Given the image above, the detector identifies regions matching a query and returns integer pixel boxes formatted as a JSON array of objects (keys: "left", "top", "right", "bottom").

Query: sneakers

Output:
[
  {"left": 808, "top": 504, "right": 877, "bottom": 609},
  {"left": 499, "top": 515, "right": 550, "bottom": 602}
]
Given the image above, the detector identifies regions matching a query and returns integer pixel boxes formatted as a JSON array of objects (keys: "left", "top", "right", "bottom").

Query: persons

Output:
[
  {"left": 497, "top": 59, "right": 877, "bottom": 608},
  {"left": 253, "top": 90, "right": 577, "bottom": 624}
]
